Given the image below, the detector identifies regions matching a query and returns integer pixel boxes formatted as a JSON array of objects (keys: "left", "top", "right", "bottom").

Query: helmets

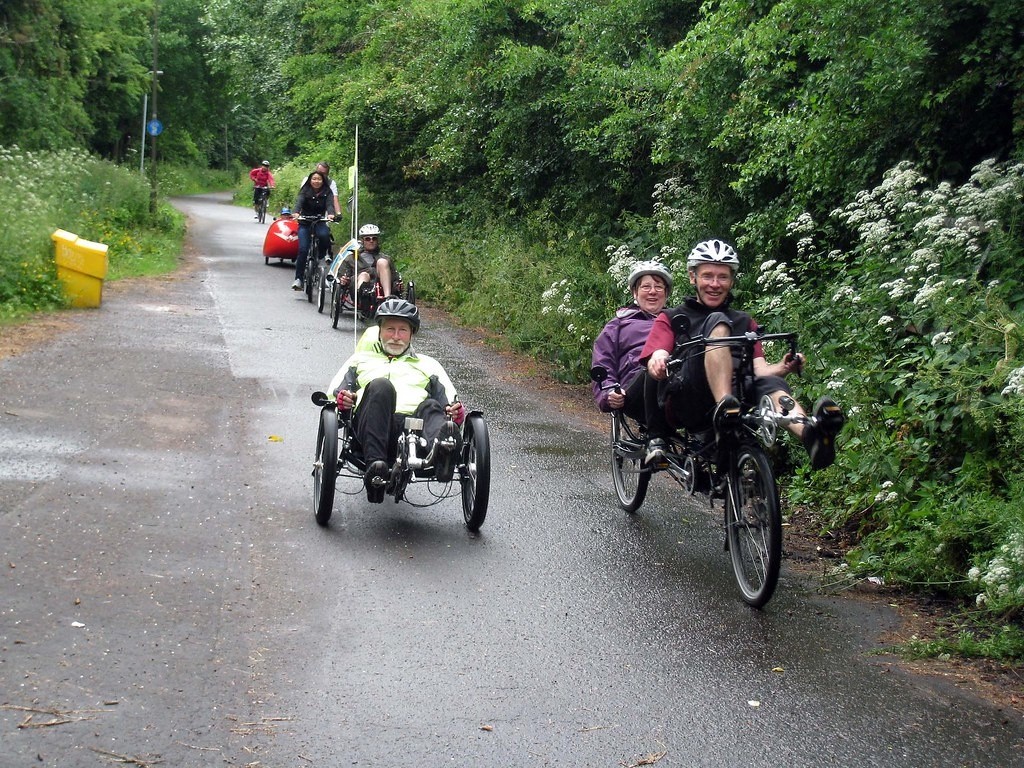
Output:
[
  {"left": 686, "top": 239, "right": 740, "bottom": 276},
  {"left": 261, "top": 160, "right": 270, "bottom": 167},
  {"left": 374, "top": 298, "right": 420, "bottom": 334},
  {"left": 627, "top": 261, "right": 672, "bottom": 293},
  {"left": 281, "top": 207, "right": 291, "bottom": 215},
  {"left": 359, "top": 223, "right": 380, "bottom": 236}
]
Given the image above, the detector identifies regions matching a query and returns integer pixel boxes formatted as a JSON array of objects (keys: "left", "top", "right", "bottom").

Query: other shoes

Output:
[
  {"left": 363, "top": 459, "right": 388, "bottom": 503},
  {"left": 254, "top": 212, "right": 259, "bottom": 218},
  {"left": 434, "top": 421, "right": 462, "bottom": 483},
  {"left": 264, "top": 198, "right": 270, "bottom": 208}
]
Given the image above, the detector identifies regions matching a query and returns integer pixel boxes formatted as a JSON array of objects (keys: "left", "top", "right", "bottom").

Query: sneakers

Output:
[
  {"left": 645, "top": 438, "right": 667, "bottom": 467},
  {"left": 324, "top": 248, "right": 333, "bottom": 262},
  {"left": 291, "top": 278, "right": 304, "bottom": 289},
  {"left": 711, "top": 394, "right": 743, "bottom": 452},
  {"left": 802, "top": 394, "right": 844, "bottom": 471}
]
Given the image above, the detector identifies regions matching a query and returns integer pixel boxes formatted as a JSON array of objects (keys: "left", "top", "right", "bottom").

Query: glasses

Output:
[
  {"left": 693, "top": 271, "right": 734, "bottom": 284},
  {"left": 636, "top": 282, "right": 668, "bottom": 292},
  {"left": 362, "top": 236, "right": 378, "bottom": 241}
]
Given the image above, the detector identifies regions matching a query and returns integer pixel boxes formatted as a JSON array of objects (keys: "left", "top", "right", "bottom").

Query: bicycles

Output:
[
  {"left": 253, "top": 185, "right": 275, "bottom": 224},
  {"left": 588, "top": 315, "right": 850, "bottom": 609},
  {"left": 288, "top": 213, "right": 341, "bottom": 304}
]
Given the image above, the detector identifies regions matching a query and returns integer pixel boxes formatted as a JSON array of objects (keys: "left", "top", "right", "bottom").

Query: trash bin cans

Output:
[{"left": 49, "top": 228, "right": 110, "bottom": 309}]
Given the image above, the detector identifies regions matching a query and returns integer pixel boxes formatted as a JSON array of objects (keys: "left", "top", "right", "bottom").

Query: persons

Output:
[
  {"left": 590, "top": 260, "right": 677, "bottom": 467},
  {"left": 325, "top": 298, "right": 466, "bottom": 503},
  {"left": 249, "top": 161, "right": 275, "bottom": 218},
  {"left": 281, "top": 207, "right": 291, "bottom": 220},
  {"left": 337, "top": 223, "right": 405, "bottom": 301},
  {"left": 639, "top": 238, "right": 844, "bottom": 472},
  {"left": 291, "top": 162, "right": 342, "bottom": 290}
]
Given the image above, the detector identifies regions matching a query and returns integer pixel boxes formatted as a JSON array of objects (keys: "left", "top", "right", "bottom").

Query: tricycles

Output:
[
  {"left": 312, "top": 365, "right": 492, "bottom": 531},
  {"left": 318, "top": 266, "right": 416, "bottom": 329}
]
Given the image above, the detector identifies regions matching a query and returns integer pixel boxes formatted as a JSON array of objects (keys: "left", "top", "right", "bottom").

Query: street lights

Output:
[{"left": 140, "top": 70, "right": 164, "bottom": 176}]
